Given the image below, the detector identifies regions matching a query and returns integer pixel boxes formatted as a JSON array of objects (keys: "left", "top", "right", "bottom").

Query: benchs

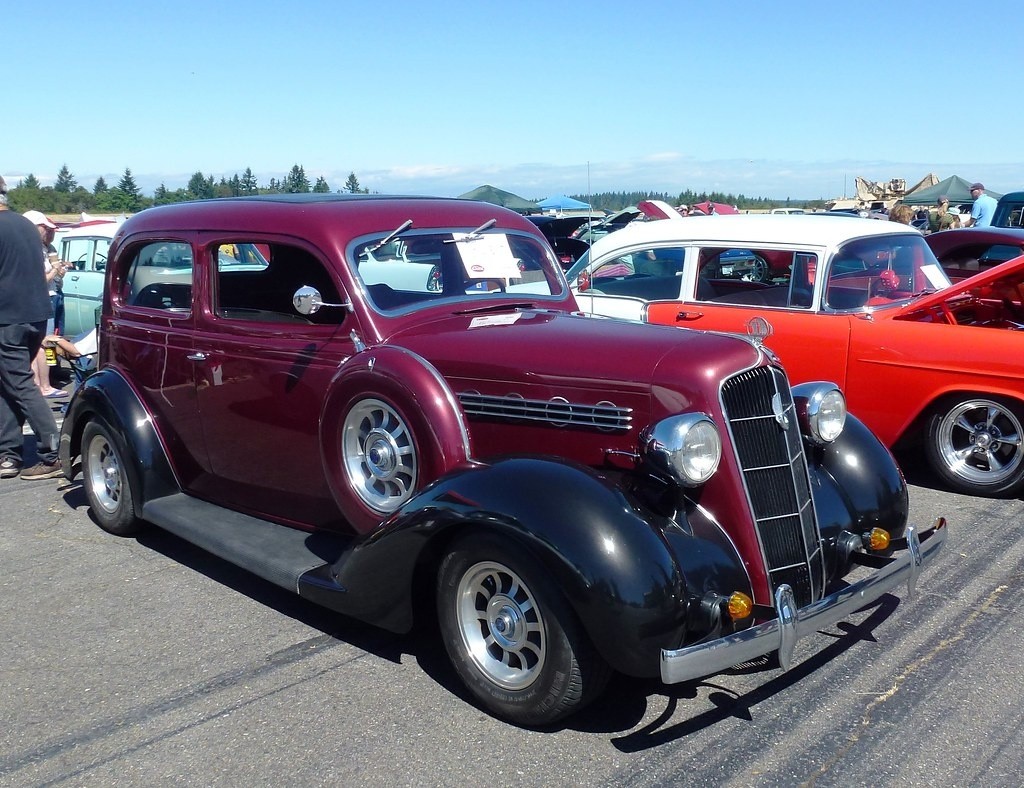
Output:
[
  {"left": 593, "top": 275, "right": 715, "bottom": 299},
  {"left": 710, "top": 286, "right": 814, "bottom": 306}
]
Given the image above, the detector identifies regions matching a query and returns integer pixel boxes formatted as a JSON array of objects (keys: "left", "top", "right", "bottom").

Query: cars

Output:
[
  {"left": 52, "top": 191, "right": 949, "bottom": 728},
  {"left": 427, "top": 191, "right": 1024, "bottom": 496},
  {"left": 50, "top": 235, "right": 320, "bottom": 368}
]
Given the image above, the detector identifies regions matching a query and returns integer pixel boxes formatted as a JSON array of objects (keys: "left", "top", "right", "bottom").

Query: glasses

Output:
[
  {"left": 39, "top": 225, "right": 50, "bottom": 230},
  {"left": 970, "top": 190, "right": 975, "bottom": 192}
]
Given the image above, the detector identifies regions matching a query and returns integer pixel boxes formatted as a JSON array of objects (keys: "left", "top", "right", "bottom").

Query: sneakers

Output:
[
  {"left": 20, "top": 460, "right": 66, "bottom": 480},
  {"left": 0, "top": 457, "right": 22, "bottom": 478}
]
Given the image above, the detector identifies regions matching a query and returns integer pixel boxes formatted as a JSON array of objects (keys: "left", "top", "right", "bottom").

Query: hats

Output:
[
  {"left": 46, "top": 218, "right": 60, "bottom": 229},
  {"left": 707, "top": 203, "right": 715, "bottom": 208},
  {"left": 0, "top": 175, "right": 7, "bottom": 195},
  {"left": 677, "top": 204, "right": 688, "bottom": 212},
  {"left": 937, "top": 196, "right": 948, "bottom": 204},
  {"left": 967, "top": 183, "right": 984, "bottom": 190},
  {"left": 946, "top": 207, "right": 960, "bottom": 216},
  {"left": 22, "top": 210, "right": 56, "bottom": 229}
]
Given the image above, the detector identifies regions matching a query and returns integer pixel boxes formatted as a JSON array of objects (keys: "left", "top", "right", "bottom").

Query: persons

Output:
[
  {"left": 1, "top": 190, "right": 68, "bottom": 483},
  {"left": 43, "top": 326, "right": 101, "bottom": 415},
  {"left": 887, "top": 194, "right": 963, "bottom": 245},
  {"left": 960, "top": 182, "right": 998, "bottom": 228}
]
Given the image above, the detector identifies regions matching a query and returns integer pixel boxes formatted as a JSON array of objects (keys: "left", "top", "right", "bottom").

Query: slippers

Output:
[{"left": 43, "top": 390, "right": 69, "bottom": 397}]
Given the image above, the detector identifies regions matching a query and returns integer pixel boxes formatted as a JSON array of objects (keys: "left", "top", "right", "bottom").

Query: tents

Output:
[
  {"left": 902, "top": 172, "right": 1004, "bottom": 206},
  {"left": 456, "top": 185, "right": 592, "bottom": 214}
]
